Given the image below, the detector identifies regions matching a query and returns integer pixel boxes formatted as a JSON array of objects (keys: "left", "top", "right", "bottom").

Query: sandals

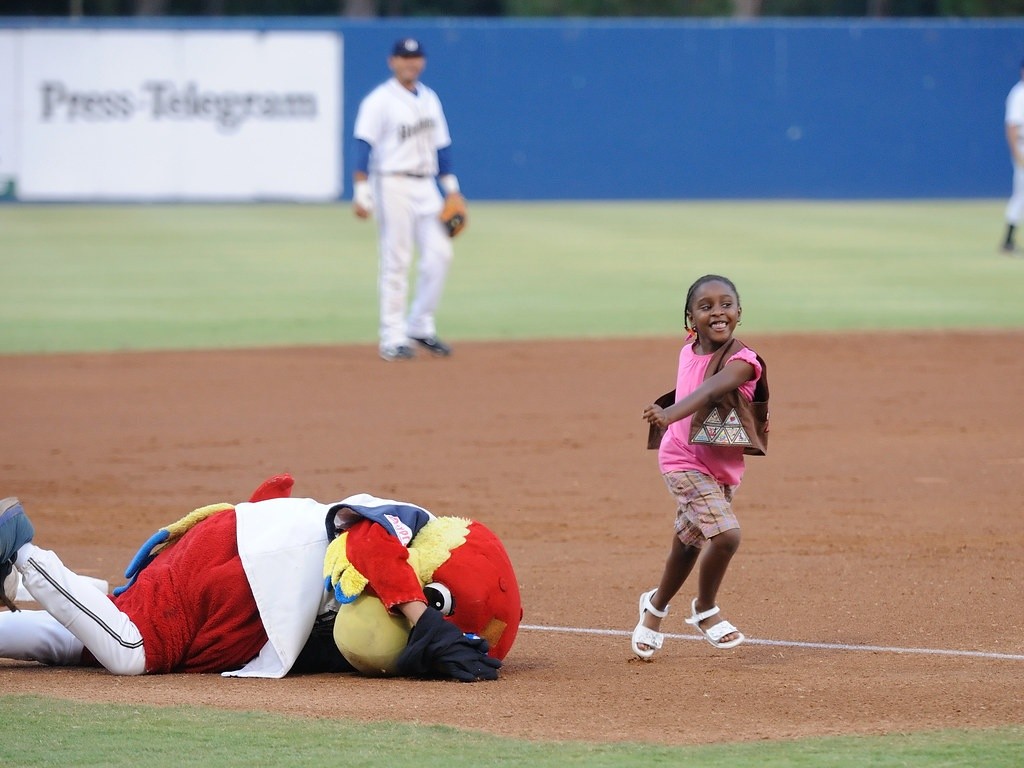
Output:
[
  {"left": 631, "top": 588, "right": 669, "bottom": 657},
  {"left": 686, "top": 597, "right": 744, "bottom": 648}
]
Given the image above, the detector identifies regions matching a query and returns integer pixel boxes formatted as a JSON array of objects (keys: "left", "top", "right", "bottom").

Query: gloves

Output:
[
  {"left": 400, "top": 606, "right": 503, "bottom": 682},
  {"left": 352, "top": 192, "right": 375, "bottom": 218}
]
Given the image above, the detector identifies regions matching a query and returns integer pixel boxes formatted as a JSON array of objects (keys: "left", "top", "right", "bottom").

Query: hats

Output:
[{"left": 391, "top": 39, "right": 427, "bottom": 56}]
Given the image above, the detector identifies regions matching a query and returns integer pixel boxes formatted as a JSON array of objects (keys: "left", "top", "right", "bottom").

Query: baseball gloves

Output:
[{"left": 438, "top": 194, "right": 468, "bottom": 236}]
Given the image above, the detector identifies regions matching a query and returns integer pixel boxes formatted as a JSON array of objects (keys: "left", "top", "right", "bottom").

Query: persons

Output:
[
  {"left": 0, "top": 474, "right": 523, "bottom": 682},
  {"left": 630, "top": 275, "right": 770, "bottom": 656},
  {"left": 351, "top": 38, "right": 467, "bottom": 360},
  {"left": 1001, "top": 57, "right": 1024, "bottom": 252}
]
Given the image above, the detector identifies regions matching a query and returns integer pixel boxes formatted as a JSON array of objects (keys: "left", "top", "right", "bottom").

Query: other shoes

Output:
[
  {"left": 410, "top": 335, "right": 451, "bottom": 358},
  {"left": 379, "top": 346, "right": 417, "bottom": 363},
  {"left": 0, "top": 496, "right": 35, "bottom": 565}
]
